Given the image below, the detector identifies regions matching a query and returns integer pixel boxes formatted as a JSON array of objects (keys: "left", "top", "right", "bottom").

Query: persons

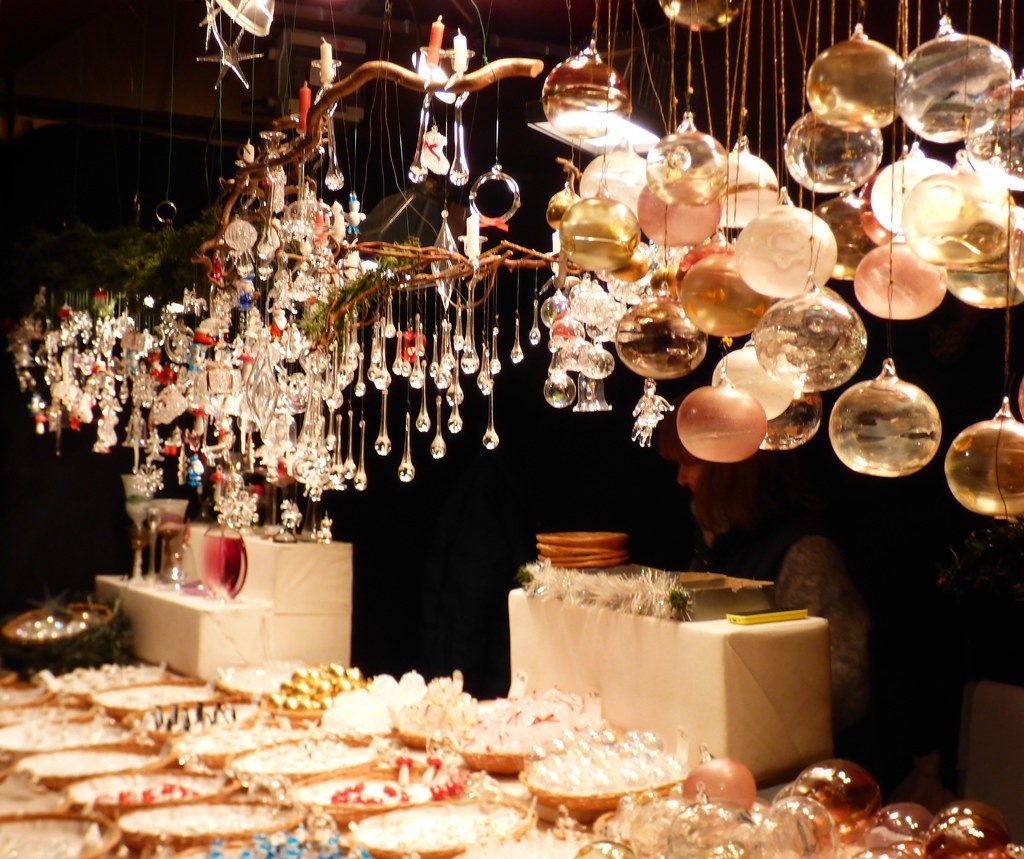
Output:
[{"left": 650, "top": 388, "right": 955, "bottom": 785}]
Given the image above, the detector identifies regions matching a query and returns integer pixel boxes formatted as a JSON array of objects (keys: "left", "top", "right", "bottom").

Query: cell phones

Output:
[{"left": 726, "top": 607, "right": 809, "bottom": 625}]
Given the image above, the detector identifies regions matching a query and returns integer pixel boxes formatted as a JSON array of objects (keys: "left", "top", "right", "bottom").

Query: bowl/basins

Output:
[
  {"left": 2, "top": 604, "right": 117, "bottom": 666},
  {"left": 0, "top": 668, "right": 771, "bottom": 859}
]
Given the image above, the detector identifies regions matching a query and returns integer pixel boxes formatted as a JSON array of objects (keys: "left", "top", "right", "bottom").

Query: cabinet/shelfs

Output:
[{"left": 95, "top": 523, "right": 352, "bottom": 677}]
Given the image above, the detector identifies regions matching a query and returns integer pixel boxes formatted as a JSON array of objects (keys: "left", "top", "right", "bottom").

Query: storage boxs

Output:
[{"left": 508, "top": 585, "right": 834, "bottom": 786}]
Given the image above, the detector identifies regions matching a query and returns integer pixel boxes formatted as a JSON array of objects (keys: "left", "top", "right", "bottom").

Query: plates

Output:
[{"left": 535, "top": 532, "right": 629, "bottom": 568}]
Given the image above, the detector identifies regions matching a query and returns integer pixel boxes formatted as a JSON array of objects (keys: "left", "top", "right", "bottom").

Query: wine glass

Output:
[{"left": 122, "top": 473, "right": 187, "bottom": 588}]
